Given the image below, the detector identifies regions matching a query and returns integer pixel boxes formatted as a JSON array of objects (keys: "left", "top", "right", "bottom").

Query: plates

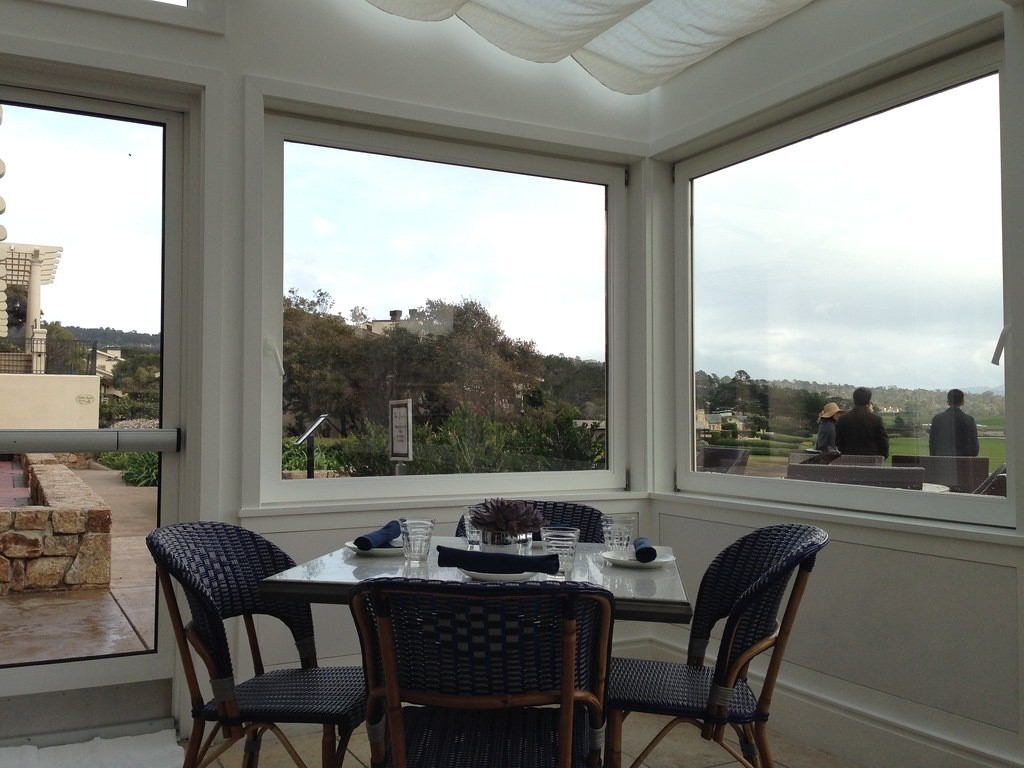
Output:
[
  {"left": 598, "top": 550, "right": 676, "bottom": 568},
  {"left": 344, "top": 539, "right": 404, "bottom": 556},
  {"left": 457, "top": 568, "right": 537, "bottom": 581}
]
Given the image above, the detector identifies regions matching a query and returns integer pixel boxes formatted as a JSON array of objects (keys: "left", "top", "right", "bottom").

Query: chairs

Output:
[
  {"left": 970, "top": 462, "right": 1006, "bottom": 495},
  {"left": 455, "top": 500, "right": 628, "bottom": 544},
  {"left": 146, "top": 520, "right": 365, "bottom": 768},
  {"left": 602, "top": 523, "right": 828, "bottom": 768},
  {"left": 349, "top": 576, "right": 613, "bottom": 768},
  {"left": 697, "top": 448, "right": 751, "bottom": 475}
]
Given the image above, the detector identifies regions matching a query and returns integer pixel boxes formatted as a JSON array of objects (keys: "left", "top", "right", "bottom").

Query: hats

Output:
[{"left": 816, "top": 402, "right": 848, "bottom": 424}]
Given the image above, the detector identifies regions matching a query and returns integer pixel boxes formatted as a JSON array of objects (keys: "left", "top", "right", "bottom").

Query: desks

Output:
[{"left": 260, "top": 534, "right": 692, "bottom": 768}]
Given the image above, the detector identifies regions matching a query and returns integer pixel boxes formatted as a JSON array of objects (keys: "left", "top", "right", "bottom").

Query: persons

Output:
[
  {"left": 815, "top": 387, "right": 890, "bottom": 460},
  {"left": 929, "top": 389, "right": 980, "bottom": 456}
]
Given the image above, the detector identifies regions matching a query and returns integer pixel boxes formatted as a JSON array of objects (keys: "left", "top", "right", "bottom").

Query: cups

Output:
[
  {"left": 400, "top": 518, "right": 434, "bottom": 560},
  {"left": 541, "top": 527, "right": 580, "bottom": 579},
  {"left": 601, "top": 515, "right": 635, "bottom": 551},
  {"left": 462, "top": 507, "right": 481, "bottom": 545}
]
